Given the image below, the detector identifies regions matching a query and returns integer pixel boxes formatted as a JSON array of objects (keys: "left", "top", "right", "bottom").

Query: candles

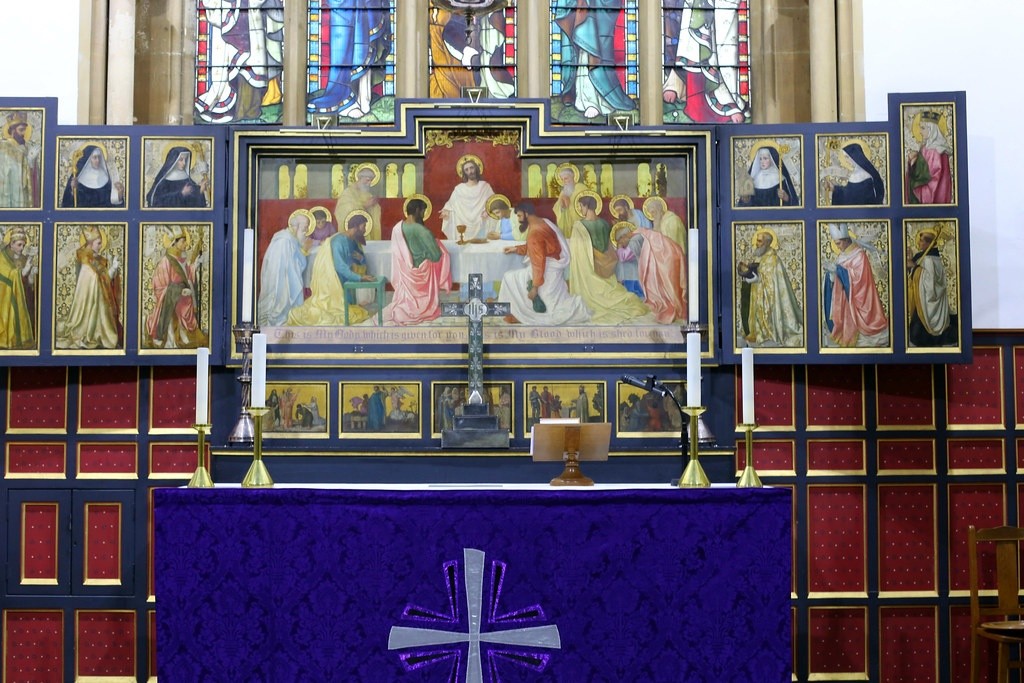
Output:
[
  {"left": 742, "top": 344, "right": 755, "bottom": 427},
  {"left": 688, "top": 332, "right": 702, "bottom": 409},
  {"left": 241, "top": 226, "right": 254, "bottom": 324},
  {"left": 195, "top": 345, "right": 210, "bottom": 425},
  {"left": 250, "top": 331, "right": 267, "bottom": 409},
  {"left": 689, "top": 227, "right": 700, "bottom": 323}
]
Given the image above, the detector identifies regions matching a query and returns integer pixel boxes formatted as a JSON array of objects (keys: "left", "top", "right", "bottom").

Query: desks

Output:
[{"left": 146, "top": 480, "right": 798, "bottom": 683}]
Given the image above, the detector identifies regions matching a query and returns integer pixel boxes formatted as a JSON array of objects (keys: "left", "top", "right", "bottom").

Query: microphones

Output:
[{"left": 621, "top": 374, "right": 667, "bottom": 397}]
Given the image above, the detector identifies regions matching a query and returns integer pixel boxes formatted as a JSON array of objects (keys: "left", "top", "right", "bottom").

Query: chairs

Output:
[{"left": 967, "top": 526, "right": 1024, "bottom": 683}]
